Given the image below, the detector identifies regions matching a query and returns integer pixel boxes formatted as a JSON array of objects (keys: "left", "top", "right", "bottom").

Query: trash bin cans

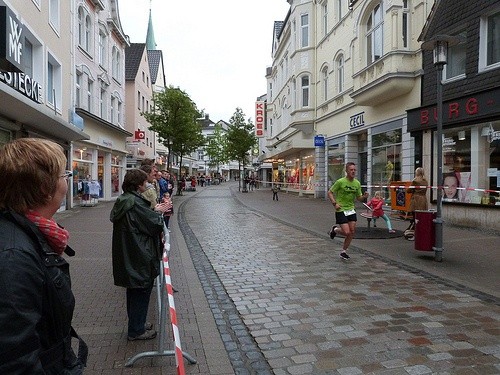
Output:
[{"left": 414, "top": 209, "right": 438, "bottom": 251}]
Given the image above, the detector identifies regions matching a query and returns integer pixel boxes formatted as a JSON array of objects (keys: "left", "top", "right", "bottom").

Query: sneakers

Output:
[
  {"left": 339, "top": 252, "right": 350, "bottom": 259},
  {"left": 330, "top": 226, "right": 337, "bottom": 239}
]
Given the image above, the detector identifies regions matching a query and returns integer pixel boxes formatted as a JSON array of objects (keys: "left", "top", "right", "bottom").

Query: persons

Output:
[
  {"left": 443, "top": 173, "right": 460, "bottom": 200},
  {"left": 329, "top": 162, "right": 370, "bottom": 261},
  {"left": 139, "top": 158, "right": 178, "bottom": 292},
  {"left": 272, "top": 183, "right": 280, "bottom": 201},
  {"left": 364, "top": 191, "right": 396, "bottom": 233},
  {"left": 0, "top": 138, "right": 90, "bottom": 375},
  {"left": 192, "top": 176, "right": 197, "bottom": 187},
  {"left": 109, "top": 168, "right": 173, "bottom": 340},
  {"left": 406, "top": 167, "right": 429, "bottom": 231}
]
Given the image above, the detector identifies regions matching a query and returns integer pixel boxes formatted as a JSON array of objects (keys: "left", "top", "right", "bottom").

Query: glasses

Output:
[{"left": 58, "top": 170, "right": 73, "bottom": 180}]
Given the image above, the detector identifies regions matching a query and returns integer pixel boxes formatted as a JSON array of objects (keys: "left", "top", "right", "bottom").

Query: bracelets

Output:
[{"left": 333, "top": 203, "right": 337, "bottom": 206}]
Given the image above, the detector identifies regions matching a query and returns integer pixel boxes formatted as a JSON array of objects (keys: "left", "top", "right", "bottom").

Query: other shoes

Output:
[
  {"left": 145, "top": 323, "right": 153, "bottom": 330},
  {"left": 389, "top": 230, "right": 396, "bottom": 233},
  {"left": 127, "top": 330, "right": 157, "bottom": 340}
]
